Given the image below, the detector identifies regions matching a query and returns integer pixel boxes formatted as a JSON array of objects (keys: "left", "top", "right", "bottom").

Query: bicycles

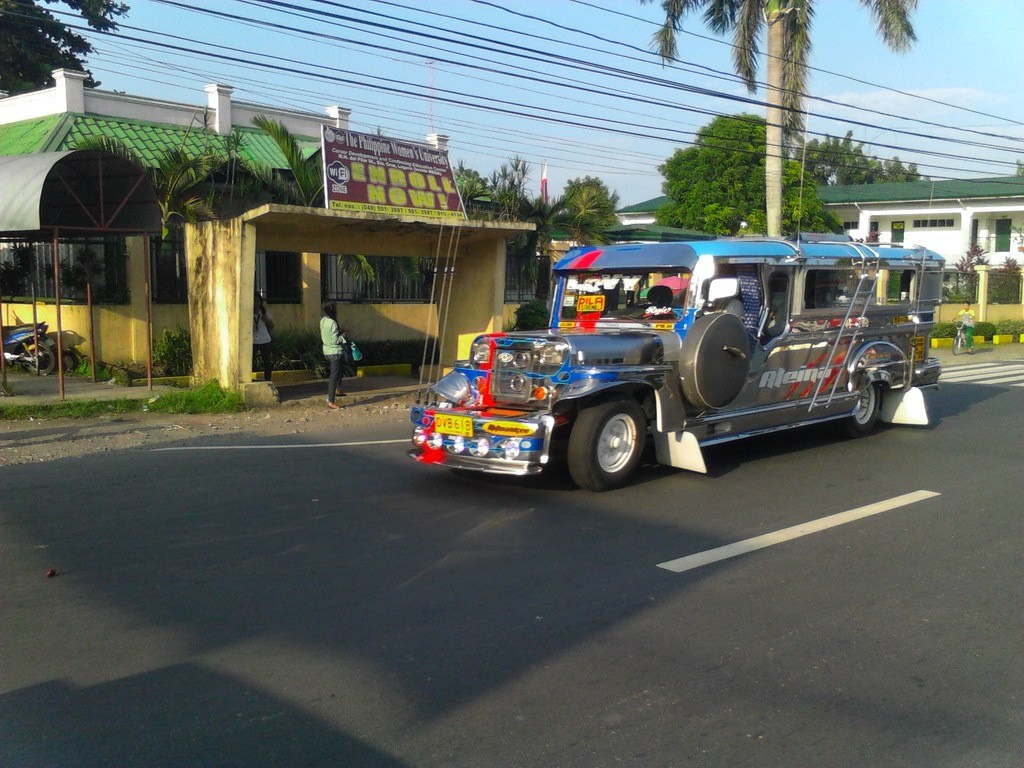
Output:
[{"left": 948, "top": 319, "right": 974, "bottom": 356}]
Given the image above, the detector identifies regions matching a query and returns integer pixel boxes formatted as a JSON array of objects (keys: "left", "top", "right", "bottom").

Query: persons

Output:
[
  {"left": 951, "top": 301, "right": 976, "bottom": 353},
  {"left": 319, "top": 300, "right": 348, "bottom": 410},
  {"left": 252, "top": 295, "right": 275, "bottom": 380}
]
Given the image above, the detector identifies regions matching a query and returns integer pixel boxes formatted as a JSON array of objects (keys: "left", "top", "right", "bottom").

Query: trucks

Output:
[{"left": 407, "top": 234, "right": 945, "bottom": 492}]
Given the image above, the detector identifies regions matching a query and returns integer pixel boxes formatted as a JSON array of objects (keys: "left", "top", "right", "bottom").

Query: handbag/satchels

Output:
[
  {"left": 341, "top": 343, "right": 356, "bottom": 368},
  {"left": 350, "top": 341, "right": 362, "bottom": 361}
]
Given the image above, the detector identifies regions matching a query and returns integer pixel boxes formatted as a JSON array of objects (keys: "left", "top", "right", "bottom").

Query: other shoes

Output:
[
  {"left": 335, "top": 389, "right": 344, "bottom": 395},
  {"left": 328, "top": 402, "right": 342, "bottom": 409}
]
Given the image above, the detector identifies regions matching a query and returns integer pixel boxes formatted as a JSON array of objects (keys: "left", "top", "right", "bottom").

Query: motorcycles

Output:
[{"left": 0, "top": 322, "right": 56, "bottom": 376}]
